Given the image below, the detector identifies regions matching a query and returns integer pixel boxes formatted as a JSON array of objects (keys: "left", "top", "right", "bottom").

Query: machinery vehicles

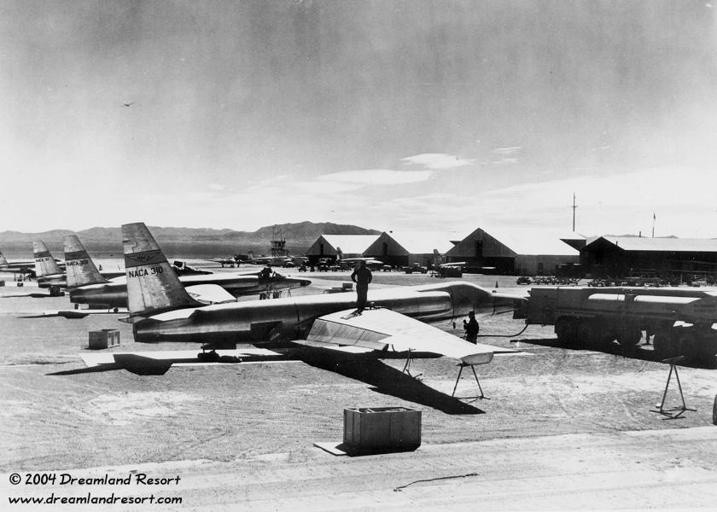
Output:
[{"left": 523, "top": 278, "right": 716, "bottom": 366}]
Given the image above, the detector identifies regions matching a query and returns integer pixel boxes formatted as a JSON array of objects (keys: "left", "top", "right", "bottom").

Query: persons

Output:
[
  {"left": 350, "top": 260, "right": 371, "bottom": 315},
  {"left": 456, "top": 311, "right": 478, "bottom": 366},
  {"left": 256, "top": 266, "right": 272, "bottom": 300}
]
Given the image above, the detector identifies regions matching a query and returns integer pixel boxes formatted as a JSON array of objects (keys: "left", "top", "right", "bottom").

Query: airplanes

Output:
[{"left": 0, "top": 223, "right": 528, "bottom": 402}]
